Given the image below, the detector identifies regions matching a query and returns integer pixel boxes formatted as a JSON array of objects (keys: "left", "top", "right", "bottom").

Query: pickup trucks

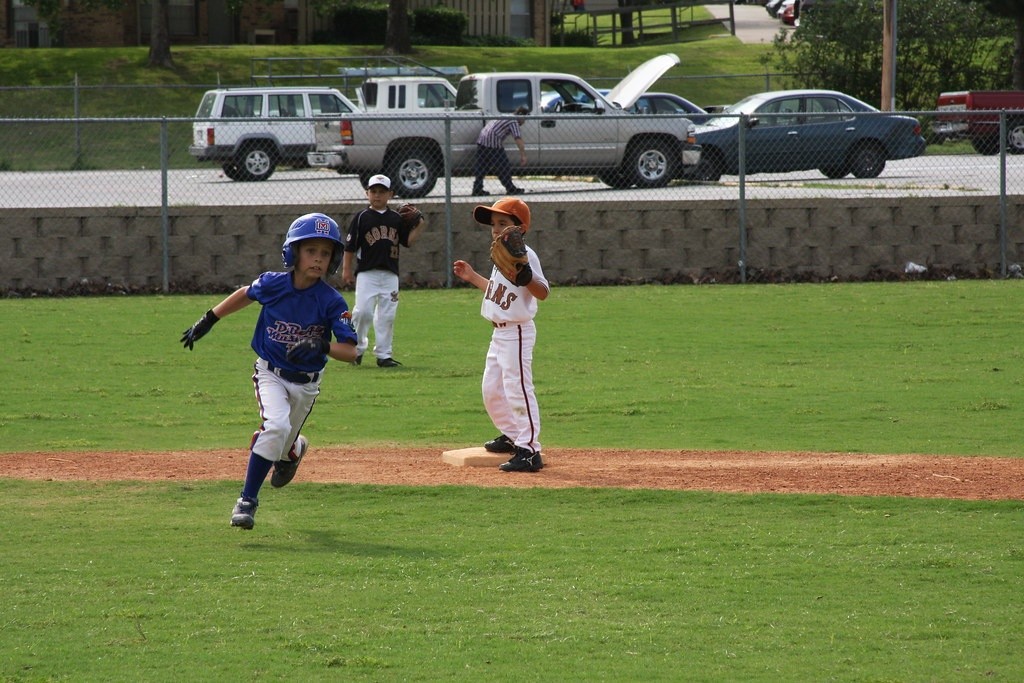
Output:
[
  {"left": 307, "top": 72, "right": 696, "bottom": 199},
  {"left": 255, "top": 77, "right": 458, "bottom": 116},
  {"left": 932, "top": 90, "right": 1024, "bottom": 155}
]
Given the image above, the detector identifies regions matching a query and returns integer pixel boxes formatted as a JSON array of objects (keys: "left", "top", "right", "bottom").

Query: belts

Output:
[{"left": 268, "top": 363, "right": 319, "bottom": 383}]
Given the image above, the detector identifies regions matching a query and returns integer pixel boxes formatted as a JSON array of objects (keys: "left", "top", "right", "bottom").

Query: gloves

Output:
[
  {"left": 180, "top": 308, "right": 220, "bottom": 350},
  {"left": 286, "top": 334, "right": 330, "bottom": 362}
]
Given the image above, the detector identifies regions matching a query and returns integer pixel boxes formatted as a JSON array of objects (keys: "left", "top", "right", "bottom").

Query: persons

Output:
[
  {"left": 453, "top": 198, "right": 550, "bottom": 472},
  {"left": 341, "top": 175, "right": 425, "bottom": 368},
  {"left": 473, "top": 106, "right": 530, "bottom": 197},
  {"left": 181, "top": 212, "right": 359, "bottom": 532}
]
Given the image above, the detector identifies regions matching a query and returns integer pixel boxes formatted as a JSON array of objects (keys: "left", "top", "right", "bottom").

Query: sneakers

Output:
[
  {"left": 484, "top": 435, "right": 523, "bottom": 452},
  {"left": 271, "top": 434, "right": 309, "bottom": 488},
  {"left": 230, "top": 492, "right": 258, "bottom": 530},
  {"left": 500, "top": 449, "right": 543, "bottom": 471}
]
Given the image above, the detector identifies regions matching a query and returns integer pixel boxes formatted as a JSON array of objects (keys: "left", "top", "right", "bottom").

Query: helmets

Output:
[{"left": 281, "top": 213, "right": 346, "bottom": 277}]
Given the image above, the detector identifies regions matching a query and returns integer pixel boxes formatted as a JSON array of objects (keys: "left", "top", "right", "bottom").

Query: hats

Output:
[
  {"left": 367, "top": 174, "right": 391, "bottom": 189},
  {"left": 473, "top": 197, "right": 531, "bottom": 232}
]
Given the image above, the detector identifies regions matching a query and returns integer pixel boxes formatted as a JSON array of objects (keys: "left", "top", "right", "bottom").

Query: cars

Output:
[
  {"left": 765, "top": 0, "right": 816, "bottom": 26},
  {"left": 511, "top": 90, "right": 731, "bottom": 114},
  {"left": 680, "top": 89, "right": 927, "bottom": 181}
]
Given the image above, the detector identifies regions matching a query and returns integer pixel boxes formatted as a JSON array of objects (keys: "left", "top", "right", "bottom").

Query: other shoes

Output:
[
  {"left": 472, "top": 189, "right": 490, "bottom": 196},
  {"left": 377, "top": 358, "right": 402, "bottom": 367},
  {"left": 353, "top": 355, "right": 362, "bottom": 365},
  {"left": 506, "top": 188, "right": 525, "bottom": 195}
]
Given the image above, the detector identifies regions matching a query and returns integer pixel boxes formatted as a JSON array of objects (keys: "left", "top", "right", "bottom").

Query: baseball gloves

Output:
[
  {"left": 490, "top": 224, "right": 533, "bottom": 287},
  {"left": 397, "top": 202, "right": 422, "bottom": 225}
]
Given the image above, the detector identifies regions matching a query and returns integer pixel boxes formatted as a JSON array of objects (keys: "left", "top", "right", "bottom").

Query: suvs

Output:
[{"left": 189, "top": 86, "right": 363, "bottom": 182}]
